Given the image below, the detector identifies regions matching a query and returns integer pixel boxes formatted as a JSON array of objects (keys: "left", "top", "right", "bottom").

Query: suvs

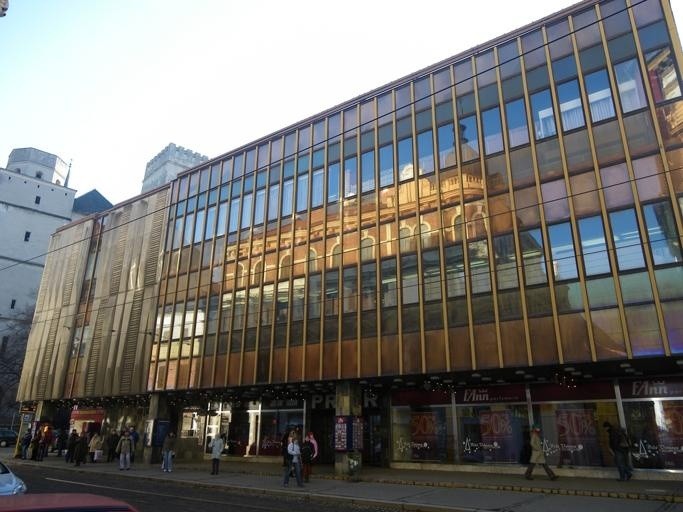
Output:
[{"left": 0, "top": 427, "right": 18, "bottom": 448}]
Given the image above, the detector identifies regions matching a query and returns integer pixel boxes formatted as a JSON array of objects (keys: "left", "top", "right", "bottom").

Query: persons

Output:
[
  {"left": 273, "top": 428, "right": 318, "bottom": 485},
  {"left": 602, "top": 419, "right": 634, "bottom": 481},
  {"left": 524, "top": 422, "right": 559, "bottom": 481},
  {"left": 207, "top": 430, "right": 223, "bottom": 475},
  {"left": 12, "top": 416, "right": 178, "bottom": 474}
]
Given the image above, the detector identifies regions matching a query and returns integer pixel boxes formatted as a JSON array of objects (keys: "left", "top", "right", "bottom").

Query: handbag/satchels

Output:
[
  {"left": 282, "top": 453, "right": 294, "bottom": 466},
  {"left": 94, "top": 450, "right": 104, "bottom": 461}
]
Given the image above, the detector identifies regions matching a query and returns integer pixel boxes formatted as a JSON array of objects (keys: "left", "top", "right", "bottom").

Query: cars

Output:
[
  {"left": 0, "top": 460, "right": 27, "bottom": 497},
  {"left": 1, "top": 490, "right": 139, "bottom": 512}
]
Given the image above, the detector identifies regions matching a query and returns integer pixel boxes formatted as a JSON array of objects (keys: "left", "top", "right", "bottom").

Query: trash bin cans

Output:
[{"left": 348, "top": 448, "right": 361, "bottom": 472}]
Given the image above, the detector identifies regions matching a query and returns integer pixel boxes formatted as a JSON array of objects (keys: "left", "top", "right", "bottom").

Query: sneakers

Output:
[
  {"left": 616, "top": 473, "right": 632, "bottom": 482},
  {"left": 551, "top": 475, "right": 559, "bottom": 481},
  {"left": 525, "top": 476, "right": 533, "bottom": 480}
]
